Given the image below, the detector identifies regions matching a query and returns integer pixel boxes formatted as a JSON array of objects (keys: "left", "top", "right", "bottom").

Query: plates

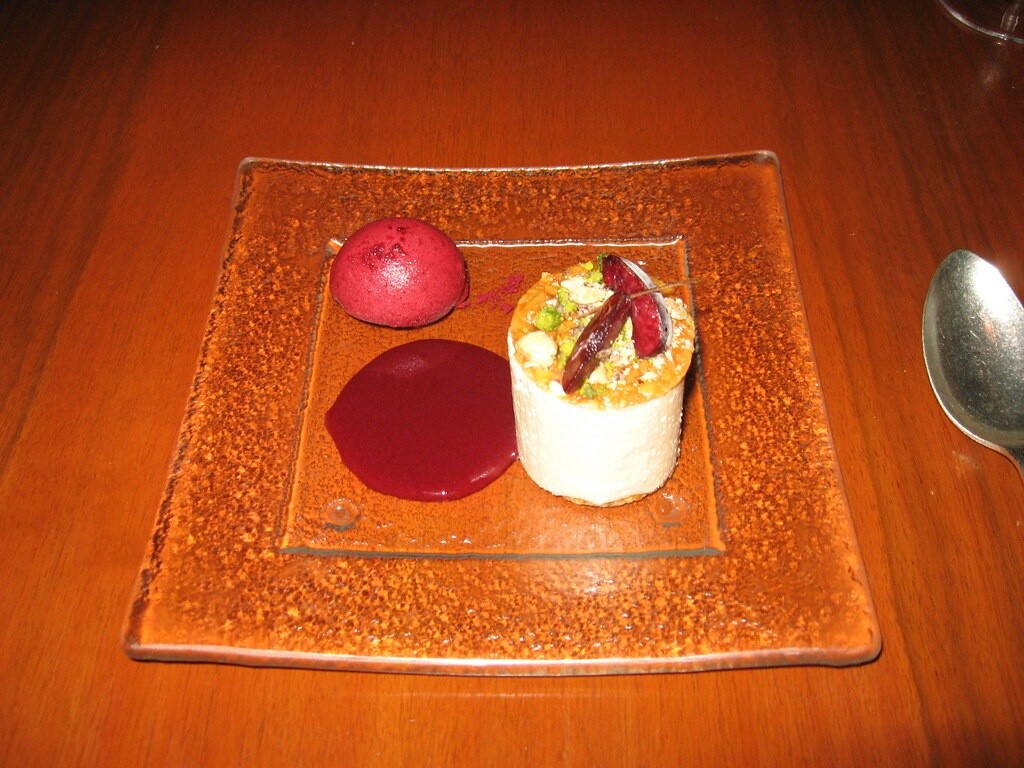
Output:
[{"left": 124, "top": 152, "right": 882, "bottom": 672}]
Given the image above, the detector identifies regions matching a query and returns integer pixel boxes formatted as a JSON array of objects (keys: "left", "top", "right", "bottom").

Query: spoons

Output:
[{"left": 920, "top": 249, "right": 1024, "bottom": 485}]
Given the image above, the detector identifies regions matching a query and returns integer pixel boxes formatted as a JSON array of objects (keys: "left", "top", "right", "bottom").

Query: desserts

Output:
[
  {"left": 328, "top": 217, "right": 470, "bottom": 329},
  {"left": 506, "top": 250, "right": 695, "bottom": 507}
]
